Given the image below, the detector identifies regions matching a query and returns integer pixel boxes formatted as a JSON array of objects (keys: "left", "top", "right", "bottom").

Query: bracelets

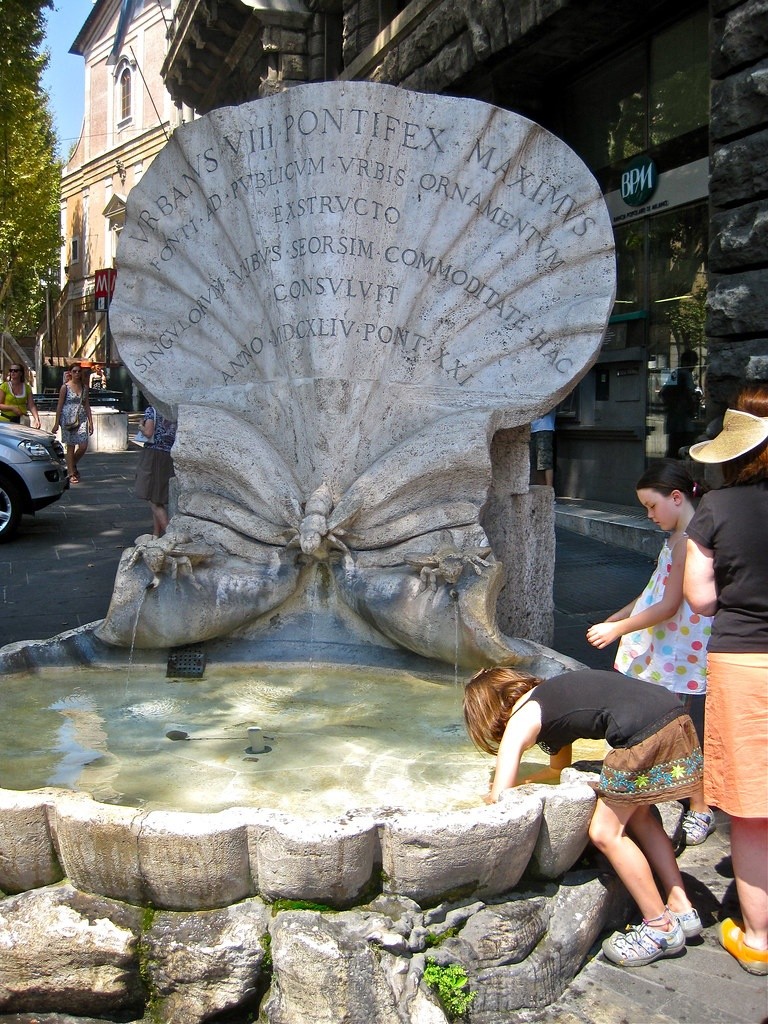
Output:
[
  {"left": 35, "top": 418, "right": 39, "bottom": 420},
  {"left": 137, "top": 425, "right": 142, "bottom": 429}
]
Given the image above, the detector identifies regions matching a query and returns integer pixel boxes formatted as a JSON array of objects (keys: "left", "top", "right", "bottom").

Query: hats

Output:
[{"left": 689, "top": 408, "right": 768, "bottom": 464}]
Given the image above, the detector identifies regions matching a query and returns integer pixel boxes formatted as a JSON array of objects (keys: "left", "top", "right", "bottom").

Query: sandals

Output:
[
  {"left": 602, "top": 918, "right": 686, "bottom": 966},
  {"left": 665, "top": 904, "right": 703, "bottom": 937},
  {"left": 681, "top": 808, "right": 716, "bottom": 845}
]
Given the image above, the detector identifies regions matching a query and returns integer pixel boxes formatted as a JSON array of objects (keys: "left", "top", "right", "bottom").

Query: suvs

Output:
[{"left": 0, "top": 421, "right": 71, "bottom": 544}]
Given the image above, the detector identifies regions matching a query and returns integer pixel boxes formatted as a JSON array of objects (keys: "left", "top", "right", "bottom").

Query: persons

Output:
[
  {"left": 530, "top": 407, "right": 556, "bottom": 504},
  {"left": 135, "top": 406, "right": 177, "bottom": 538},
  {"left": 587, "top": 349, "right": 768, "bottom": 976},
  {"left": 462, "top": 666, "right": 703, "bottom": 966},
  {"left": 64, "top": 365, "right": 106, "bottom": 390},
  {"left": 52, "top": 363, "right": 93, "bottom": 484},
  {"left": 0, "top": 363, "right": 41, "bottom": 429}
]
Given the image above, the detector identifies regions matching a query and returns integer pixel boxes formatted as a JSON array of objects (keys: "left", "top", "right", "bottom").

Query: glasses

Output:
[
  {"left": 71, "top": 370, "right": 81, "bottom": 373},
  {"left": 9, "top": 369, "right": 22, "bottom": 372}
]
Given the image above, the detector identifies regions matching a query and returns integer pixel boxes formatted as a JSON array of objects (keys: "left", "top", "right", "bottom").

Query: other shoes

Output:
[
  {"left": 718, "top": 917, "right": 768, "bottom": 975},
  {"left": 68, "top": 466, "right": 80, "bottom": 479},
  {"left": 68, "top": 474, "right": 78, "bottom": 482}
]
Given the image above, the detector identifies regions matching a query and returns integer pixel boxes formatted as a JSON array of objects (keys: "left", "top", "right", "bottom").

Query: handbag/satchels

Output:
[
  {"left": 64, "top": 415, "right": 79, "bottom": 430},
  {"left": 134, "top": 407, "right": 156, "bottom": 444},
  {"left": 20, "top": 414, "right": 30, "bottom": 427},
  {"left": 2, "top": 413, "right": 20, "bottom": 423}
]
